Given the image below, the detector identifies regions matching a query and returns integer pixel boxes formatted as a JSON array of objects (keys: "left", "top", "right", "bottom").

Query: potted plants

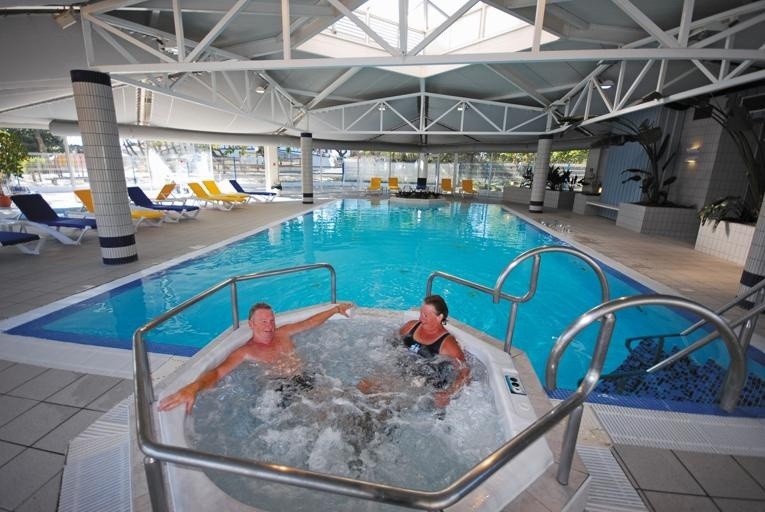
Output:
[
  {"left": 269, "top": 184, "right": 283, "bottom": 198},
  {"left": 0, "top": 130, "right": 28, "bottom": 207}
]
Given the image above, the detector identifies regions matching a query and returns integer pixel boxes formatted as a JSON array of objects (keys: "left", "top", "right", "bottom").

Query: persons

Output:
[
  {"left": 157, "top": 303, "right": 354, "bottom": 426},
  {"left": 356, "top": 295, "right": 470, "bottom": 408}
]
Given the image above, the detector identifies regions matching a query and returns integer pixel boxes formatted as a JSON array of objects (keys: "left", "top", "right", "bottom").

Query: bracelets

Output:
[{"left": 335, "top": 306, "right": 340, "bottom": 313}]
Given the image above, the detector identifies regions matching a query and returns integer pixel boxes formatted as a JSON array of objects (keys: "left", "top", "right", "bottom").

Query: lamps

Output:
[
  {"left": 52, "top": 8, "right": 86, "bottom": 32},
  {"left": 254, "top": 80, "right": 270, "bottom": 95},
  {"left": 156, "top": 37, "right": 179, "bottom": 55}
]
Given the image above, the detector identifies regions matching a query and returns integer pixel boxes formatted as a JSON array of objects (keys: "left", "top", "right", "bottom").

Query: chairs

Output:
[
  {"left": 0, "top": 219, "right": 45, "bottom": 255},
  {"left": 438, "top": 178, "right": 455, "bottom": 195},
  {"left": 9, "top": 189, "right": 92, "bottom": 245},
  {"left": 63, "top": 180, "right": 276, "bottom": 230},
  {"left": 386, "top": 177, "right": 401, "bottom": 193},
  {"left": 366, "top": 176, "right": 384, "bottom": 195},
  {"left": 459, "top": 179, "right": 479, "bottom": 199},
  {"left": 416, "top": 177, "right": 430, "bottom": 193}
]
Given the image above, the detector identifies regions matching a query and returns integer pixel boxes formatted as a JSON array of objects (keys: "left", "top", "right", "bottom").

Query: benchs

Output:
[{"left": 587, "top": 200, "right": 620, "bottom": 213}]
